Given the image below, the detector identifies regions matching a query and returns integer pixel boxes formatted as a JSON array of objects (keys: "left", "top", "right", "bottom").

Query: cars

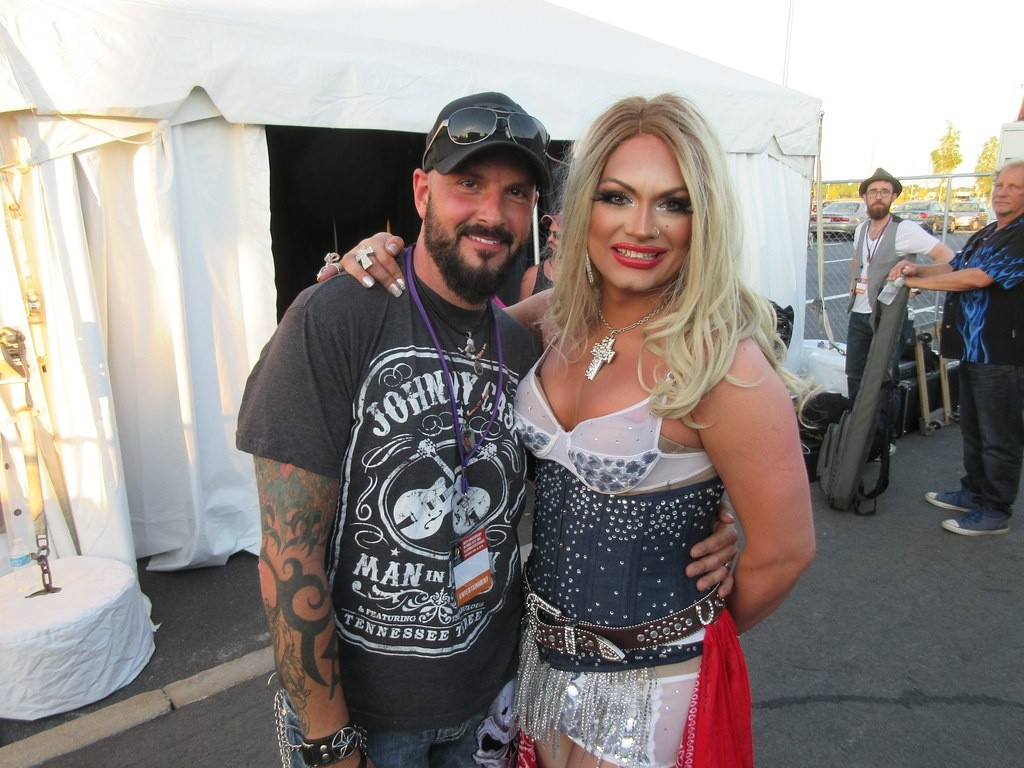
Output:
[
  {"left": 949, "top": 201, "right": 988, "bottom": 230},
  {"left": 892, "top": 199, "right": 954, "bottom": 236},
  {"left": 810, "top": 200, "right": 870, "bottom": 238}
]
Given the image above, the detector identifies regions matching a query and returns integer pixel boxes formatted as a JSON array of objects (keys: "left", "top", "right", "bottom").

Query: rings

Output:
[
  {"left": 724, "top": 563, "right": 731, "bottom": 572},
  {"left": 317, "top": 253, "right": 343, "bottom": 278},
  {"left": 355, "top": 246, "right": 374, "bottom": 269}
]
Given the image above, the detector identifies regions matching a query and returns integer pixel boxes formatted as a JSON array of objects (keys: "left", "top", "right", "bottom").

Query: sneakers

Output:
[
  {"left": 924, "top": 487, "right": 980, "bottom": 513},
  {"left": 941, "top": 506, "right": 1010, "bottom": 537}
]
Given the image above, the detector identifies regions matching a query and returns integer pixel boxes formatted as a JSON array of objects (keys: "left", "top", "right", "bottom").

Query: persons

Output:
[
  {"left": 845, "top": 169, "right": 954, "bottom": 456},
  {"left": 318, "top": 92, "right": 815, "bottom": 768},
  {"left": 885, "top": 163, "right": 1024, "bottom": 536},
  {"left": 237, "top": 92, "right": 738, "bottom": 768},
  {"left": 520, "top": 214, "right": 562, "bottom": 303}
]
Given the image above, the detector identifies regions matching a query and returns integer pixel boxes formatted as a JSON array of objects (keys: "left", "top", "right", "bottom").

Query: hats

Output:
[
  {"left": 859, "top": 168, "right": 903, "bottom": 198},
  {"left": 422, "top": 92, "right": 553, "bottom": 192}
]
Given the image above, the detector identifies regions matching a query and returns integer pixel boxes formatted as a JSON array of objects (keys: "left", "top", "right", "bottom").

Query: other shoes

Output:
[{"left": 874, "top": 444, "right": 897, "bottom": 461}]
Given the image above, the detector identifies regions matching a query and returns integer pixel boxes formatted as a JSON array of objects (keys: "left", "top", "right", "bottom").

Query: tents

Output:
[{"left": 0, "top": 0, "right": 825, "bottom": 585}]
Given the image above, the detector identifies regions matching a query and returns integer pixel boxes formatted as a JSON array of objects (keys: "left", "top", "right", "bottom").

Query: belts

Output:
[{"left": 521, "top": 562, "right": 726, "bottom": 661}]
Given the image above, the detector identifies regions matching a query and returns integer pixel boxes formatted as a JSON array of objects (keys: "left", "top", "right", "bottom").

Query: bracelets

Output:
[
  {"left": 358, "top": 750, "right": 367, "bottom": 768},
  {"left": 299, "top": 721, "right": 367, "bottom": 768}
]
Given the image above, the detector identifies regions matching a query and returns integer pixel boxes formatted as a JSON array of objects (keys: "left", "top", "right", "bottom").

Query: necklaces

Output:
[
  {"left": 585, "top": 294, "right": 671, "bottom": 380},
  {"left": 410, "top": 259, "right": 491, "bottom": 377}
]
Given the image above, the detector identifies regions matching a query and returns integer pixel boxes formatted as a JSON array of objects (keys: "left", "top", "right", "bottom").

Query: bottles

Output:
[
  {"left": 9, "top": 539, "right": 33, "bottom": 571},
  {"left": 877, "top": 266, "right": 911, "bottom": 306}
]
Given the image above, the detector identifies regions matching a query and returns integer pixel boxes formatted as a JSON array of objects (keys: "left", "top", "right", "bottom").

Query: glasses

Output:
[
  {"left": 865, "top": 189, "right": 893, "bottom": 197},
  {"left": 423, "top": 107, "right": 550, "bottom": 169}
]
